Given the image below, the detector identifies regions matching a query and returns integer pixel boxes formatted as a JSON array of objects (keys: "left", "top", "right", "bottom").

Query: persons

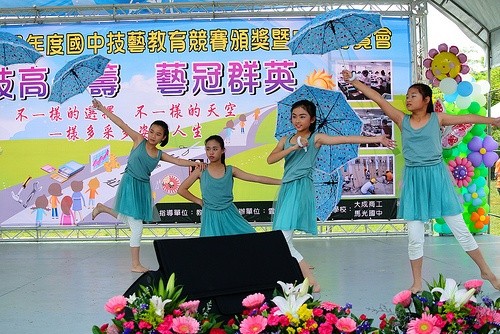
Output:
[
  {"left": 177, "top": 135, "right": 283, "bottom": 238},
  {"left": 342, "top": 169, "right": 393, "bottom": 196},
  {"left": 341, "top": 68, "right": 500, "bottom": 294},
  {"left": 351, "top": 68, "right": 391, "bottom": 100},
  {"left": 494, "top": 156, "right": 500, "bottom": 194},
  {"left": 267, "top": 99, "right": 398, "bottom": 293},
  {"left": 92, "top": 97, "right": 207, "bottom": 272},
  {"left": 364, "top": 119, "right": 392, "bottom": 147}
]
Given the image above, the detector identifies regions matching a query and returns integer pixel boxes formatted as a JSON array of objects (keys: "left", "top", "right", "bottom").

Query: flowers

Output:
[
  {"left": 90, "top": 271, "right": 226, "bottom": 334},
  {"left": 222, "top": 278, "right": 381, "bottom": 334},
  {"left": 378, "top": 272, "right": 500, "bottom": 334}
]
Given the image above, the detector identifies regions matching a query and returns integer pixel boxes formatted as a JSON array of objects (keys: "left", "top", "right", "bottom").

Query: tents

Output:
[{"left": 486, "top": 102, "right": 500, "bottom": 120}]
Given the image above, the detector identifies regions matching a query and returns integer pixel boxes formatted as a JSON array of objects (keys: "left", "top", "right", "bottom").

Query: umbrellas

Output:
[
  {"left": 273, "top": 84, "right": 364, "bottom": 175},
  {"left": 48, "top": 54, "right": 111, "bottom": 108},
  {"left": 0, "top": 32, "right": 43, "bottom": 67},
  {"left": 284, "top": 9, "right": 383, "bottom": 81},
  {"left": 312, "top": 168, "right": 345, "bottom": 223}
]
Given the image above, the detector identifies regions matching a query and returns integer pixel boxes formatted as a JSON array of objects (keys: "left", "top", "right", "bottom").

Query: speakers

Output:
[{"left": 152, "top": 229, "right": 304, "bottom": 327}]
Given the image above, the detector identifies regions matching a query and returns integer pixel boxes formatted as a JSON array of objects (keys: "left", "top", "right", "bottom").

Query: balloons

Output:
[{"left": 433, "top": 74, "right": 499, "bottom": 237}]
